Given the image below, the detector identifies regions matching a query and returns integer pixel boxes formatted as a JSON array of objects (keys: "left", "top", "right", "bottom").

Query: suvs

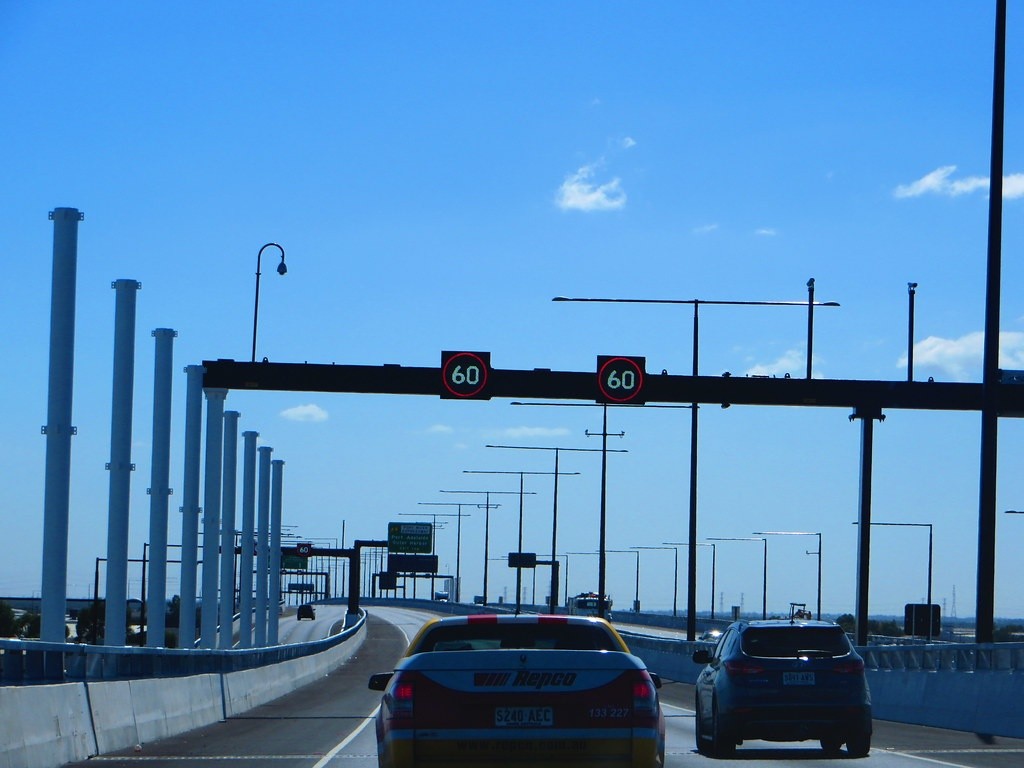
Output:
[
  {"left": 296, "top": 604, "right": 316, "bottom": 621},
  {"left": 692, "top": 618, "right": 875, "bottom": 759}
]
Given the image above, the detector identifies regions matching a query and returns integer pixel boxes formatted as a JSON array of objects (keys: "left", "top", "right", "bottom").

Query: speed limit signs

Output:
[
  {"left": 597, "top": 355, "right": 645, "bottom": 406},
  {"left": 441, "top": 351, "right": 491, "bottom": 401}
]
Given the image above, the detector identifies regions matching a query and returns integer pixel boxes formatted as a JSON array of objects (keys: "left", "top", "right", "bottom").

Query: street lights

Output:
[{"left": 251, "top": 243, "right": 288, "bottom": 363}]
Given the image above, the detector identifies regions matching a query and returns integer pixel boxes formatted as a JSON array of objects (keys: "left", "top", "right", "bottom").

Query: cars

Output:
[{"left": 369, "top": 612, "right": 670, "bottom": 768}]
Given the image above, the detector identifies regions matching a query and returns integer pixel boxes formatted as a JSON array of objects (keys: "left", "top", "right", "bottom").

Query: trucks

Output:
[
  {"left": 569, "top": 592, "right": 612, "bottom": 623},
  {"left": 435, "top": 591, "right": 450, "bottom": 602},
  {"left": 473, "top": 595, "right": 488, "bottom": 606}
]
